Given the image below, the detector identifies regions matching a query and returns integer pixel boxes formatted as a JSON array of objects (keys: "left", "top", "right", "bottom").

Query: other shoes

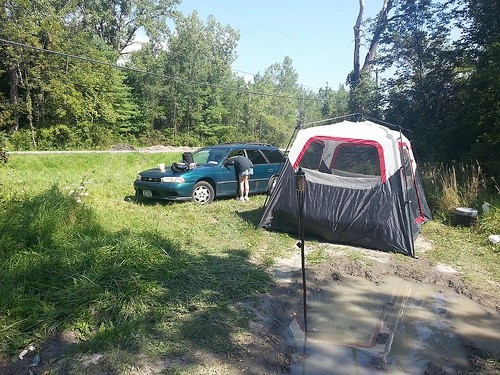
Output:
[
  {"left": 240, "top": 196, "right": 244, "bottom": 201},
  {"left": 244, "top": 196, "right": 249, "bottom": 201}
]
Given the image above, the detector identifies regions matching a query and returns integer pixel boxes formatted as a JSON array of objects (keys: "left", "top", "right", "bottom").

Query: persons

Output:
[{"left": 226, "top": 155, "right": 254, "bottom": 202}]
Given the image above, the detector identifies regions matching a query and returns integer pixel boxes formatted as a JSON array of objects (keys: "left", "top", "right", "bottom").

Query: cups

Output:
[{"left": 158, "top": 162, "right": 166, "bottom": 172}]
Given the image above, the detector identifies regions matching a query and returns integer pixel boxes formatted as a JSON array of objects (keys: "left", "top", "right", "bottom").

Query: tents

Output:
[{"left": 255, "top": 118, "right": 435, "bottom": 260}]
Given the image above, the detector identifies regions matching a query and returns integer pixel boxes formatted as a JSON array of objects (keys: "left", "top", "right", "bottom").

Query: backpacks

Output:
[
  {"left": 182, "top": 152, "right": 194, "bottom": 163},
  {"left": 171, "top": 163, "right": 189, "bottom": 172}
]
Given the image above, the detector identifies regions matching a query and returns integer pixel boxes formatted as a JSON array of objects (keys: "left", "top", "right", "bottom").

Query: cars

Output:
[{"left": 134, "top": 143, "right": 289, "bottom": 207}]
